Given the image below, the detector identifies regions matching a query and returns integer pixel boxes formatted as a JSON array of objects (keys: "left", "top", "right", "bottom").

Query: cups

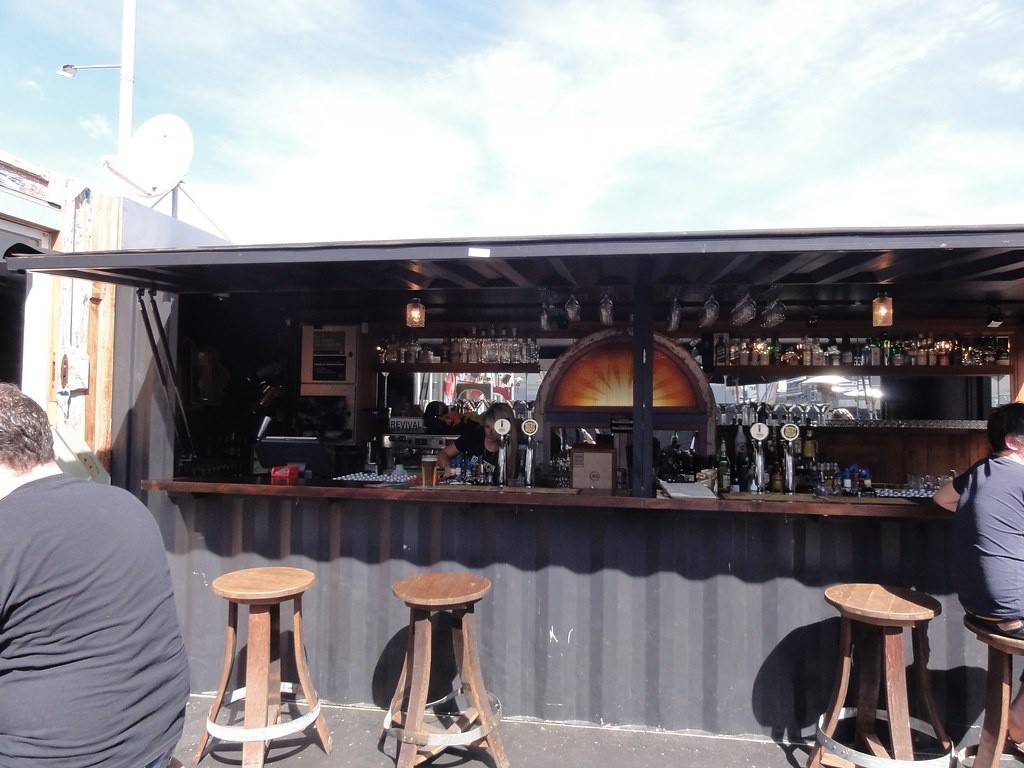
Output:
[{"left": 422, "top": 455, "right": 438, "bottom": 488}]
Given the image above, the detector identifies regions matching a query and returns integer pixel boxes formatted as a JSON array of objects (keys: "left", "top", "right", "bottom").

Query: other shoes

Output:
[{"left": 1005, "top": 729, "right": 1024, "bottom": 754}]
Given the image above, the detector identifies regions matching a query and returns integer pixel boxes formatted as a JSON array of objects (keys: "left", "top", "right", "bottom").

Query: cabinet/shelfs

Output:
[
  {"left": 377, "top": 329, "right": 1012, "bottom": 488},
  {"left": 298, "top": 322, "right": 376, "bottom": 447}
]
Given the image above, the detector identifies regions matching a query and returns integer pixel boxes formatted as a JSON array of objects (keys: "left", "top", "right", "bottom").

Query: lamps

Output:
[
  {"left": 405, "top": 290, "right": 425, "bottom": 328},
  {"left": 871, "top": 286, "right": 893, "bottom": 326}
]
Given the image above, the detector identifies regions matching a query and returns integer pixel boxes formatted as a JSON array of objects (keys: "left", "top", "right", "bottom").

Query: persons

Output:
[
  {"left": 933, "top": 402, "right": 1024, "bottom": 751},
  {"left": 438, "top": 402, "right": 519, "bottom": 486},
  {"left": 0, "top": 381, "right": 191, "bottom": 768}
]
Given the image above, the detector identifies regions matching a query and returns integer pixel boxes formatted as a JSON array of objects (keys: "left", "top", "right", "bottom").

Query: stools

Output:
[
  {"left": 376, "top": 571, "right": 511, "bottom": 768},
  {"left": 192, "top": 566, "right": 333, "bottom": 768},
  {"left": 805, "top": 582, "right": 958, "bottom": 767},
  {"left": 955, "top": 614, "right": 1023, "bottom": 767}
]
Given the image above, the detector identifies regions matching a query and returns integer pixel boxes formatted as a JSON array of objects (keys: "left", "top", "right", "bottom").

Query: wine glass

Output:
[
  {"left": 666, "top": 298, "right": 680, "bottom": 331},
  {"left": 730, "top": 284, "right": 757, "bottom": 327},
  {"left": 565, "top": 287, "right": 580, "bottom": 321},
  {"left": 600, "top": 287, "right": 615, "bottom": 325},
  {"left": 761, "top": 285, "right": 787, "bottom": 328},
  {"left": 538, "top": 288, "right": 553, "bottom": 331},
  {"left": 698, "top": 293, "right": 719, "bottom": 328}
]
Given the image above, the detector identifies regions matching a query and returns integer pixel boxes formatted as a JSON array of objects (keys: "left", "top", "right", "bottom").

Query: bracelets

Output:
[{"left": 443, "top": 464, "right": 450, "bottom": 467}]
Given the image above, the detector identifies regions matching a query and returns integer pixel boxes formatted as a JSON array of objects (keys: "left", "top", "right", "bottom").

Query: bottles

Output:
[
  {"left": 391, "top": 464, "right": 408, "bottom": 475},
  {"left": 524, "top": 436, "right": 534, "bottom": 488},
  {"left": 378, "top": 334, "right": 434, "bottom": 363},
  {"left": 713, "top": 333, "right": 1010, "bottom": 366},
  {"left": 447, "top": 464, "right": 494, "bottom": 486},
  {"left": 497, "top": 434, "right": 508, "bottom": 486},
  {"left": 441, "top": 327, "right": 540, "bottom": 364},
  {"left": 717, "top": 426, "right": 877, "bottom": 497}
]
